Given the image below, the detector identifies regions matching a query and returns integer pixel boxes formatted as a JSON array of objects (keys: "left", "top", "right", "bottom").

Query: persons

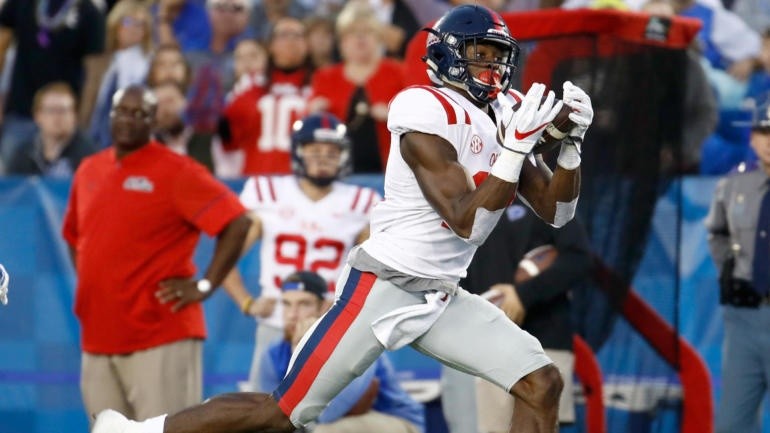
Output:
[
  {"left": 259, "top": 270, "right": 334, "bottom": 395},
  {"left": 92, "top": 5, "right": 594, "bottom": 433},
  {"left": 221, "top": 114, "right": 384, "bottom": 392},
  {"left": 0, "top": 0, "right": 770, "bottom": 433},
  {"left": 64, "top": 85, "right": 253, "bottom": 428}
]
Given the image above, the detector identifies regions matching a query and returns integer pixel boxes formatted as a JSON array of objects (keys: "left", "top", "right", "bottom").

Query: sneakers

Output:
[{"left": 89, "top": 409, "right": 132, "bottom": 433}]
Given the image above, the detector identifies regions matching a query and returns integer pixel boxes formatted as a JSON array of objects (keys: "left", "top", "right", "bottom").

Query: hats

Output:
[{"left": 731, "top": 97, "right": 770, "bottom": 128}]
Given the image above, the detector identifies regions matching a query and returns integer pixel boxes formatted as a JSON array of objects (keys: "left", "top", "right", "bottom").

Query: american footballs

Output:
[{"left": 496, "top": 96, "right": 575, "bottom": 156}]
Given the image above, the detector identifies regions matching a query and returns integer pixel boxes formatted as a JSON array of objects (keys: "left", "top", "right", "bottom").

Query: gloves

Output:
[
  {"left": 497, "top": 83, "right": 563, "bottom": 153},
  {"left": 563, "top": 80, "right": 594, "bottom": 144}
]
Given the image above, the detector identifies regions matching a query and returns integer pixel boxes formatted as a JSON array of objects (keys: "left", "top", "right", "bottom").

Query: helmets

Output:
[
  {"left": 422, "top": 5, "right": 520, "bottom": 109},
  {"left": 290, "top": 112, "right": 352, "bottom": 185}
]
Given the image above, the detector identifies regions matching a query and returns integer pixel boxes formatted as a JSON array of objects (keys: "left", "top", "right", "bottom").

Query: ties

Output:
[{"left": 753, "top": 177, "right": 770, "bottom": 298}]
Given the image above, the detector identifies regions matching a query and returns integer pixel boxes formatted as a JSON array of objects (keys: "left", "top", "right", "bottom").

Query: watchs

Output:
[{"left": 196, "top": 279, "right": 213, "bottom": 294}]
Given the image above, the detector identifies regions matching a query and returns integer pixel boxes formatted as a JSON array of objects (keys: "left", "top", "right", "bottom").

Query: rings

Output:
[{"left": 175, "top": 291, "right": 184, "bottom": 298}]
